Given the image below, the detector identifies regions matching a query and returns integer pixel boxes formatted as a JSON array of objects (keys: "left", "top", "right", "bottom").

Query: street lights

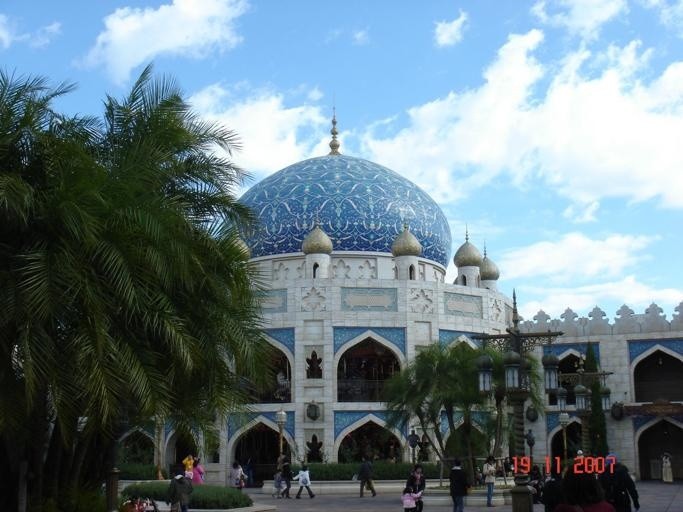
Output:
[
  {"left": 558, "top": 413, "right": 568, "bottom": 462},
  {"left": 526, "top": 428, "right": 535, "bottom": 470},
  {"left": 276, "top": 407, "right": 287, "bottom": 472},
  {"left": 557, "top": 354, "right": 613, "bottom": 457},
  {"left": 154, "top": 414, "right": 167, "bottom": 479},
  {"left": 470, "top": 284, "right": 564, "bottom": 512}
]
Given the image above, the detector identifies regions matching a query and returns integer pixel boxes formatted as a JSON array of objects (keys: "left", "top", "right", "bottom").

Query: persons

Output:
[
  {"left": 190, "top": 458, "right": 205, "bottom": 484},
  {"left": 164, "top": 468, "right": 193, "bottom": 512},
  {"left": 227, "top": 462, "right": 248, "bottom": 491},
  {"left": 449, "top": 459, "right": 470, "bottom": 512},
  {"left": 357, "top": 456, "right": 376, "bottom": 498},
  {"left": 398, "top": 486, "right": 422, "bottom": 512},
  {"left": 182, "top": 454, "right": 192, "bottom": 479},
  {"left": 270, "top": 454, "right": 315, "bottom": 499},
  {"left": 404, "top": 465, "right": 425, "bottom": 512},
  {"left": 474, "top": 454, "right": 640, "bottom": 512}
]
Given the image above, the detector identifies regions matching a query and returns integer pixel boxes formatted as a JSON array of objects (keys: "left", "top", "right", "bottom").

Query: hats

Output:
[{"left": 488, "top": 456, "right": 496, "bottom": 462}]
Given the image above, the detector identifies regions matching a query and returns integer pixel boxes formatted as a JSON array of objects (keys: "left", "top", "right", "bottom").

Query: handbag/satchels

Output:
[
  {"left": 302, "top": 479, "right": 307, "bottom": 484},
  {"left": 171, "top": 500, "right": 181, "bottom": 512},
  {"left": 479, "top": 473, "right": 485, "bottom": 485},
  {"left": 240, "top": 475, "right": 246, "bottom": 488}
]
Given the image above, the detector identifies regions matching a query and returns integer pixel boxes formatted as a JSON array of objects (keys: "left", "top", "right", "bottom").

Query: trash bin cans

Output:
[{"left": 649, "top": 458, "right": 663, "bottom": 480}]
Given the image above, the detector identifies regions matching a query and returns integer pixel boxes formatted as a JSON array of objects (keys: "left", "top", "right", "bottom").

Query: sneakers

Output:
[
  {"left": 272, "top": 494, "right": 315, "bottom": 499},
  {"left": 487, "top": 504, "right": 496, "bottom": 507}
]
